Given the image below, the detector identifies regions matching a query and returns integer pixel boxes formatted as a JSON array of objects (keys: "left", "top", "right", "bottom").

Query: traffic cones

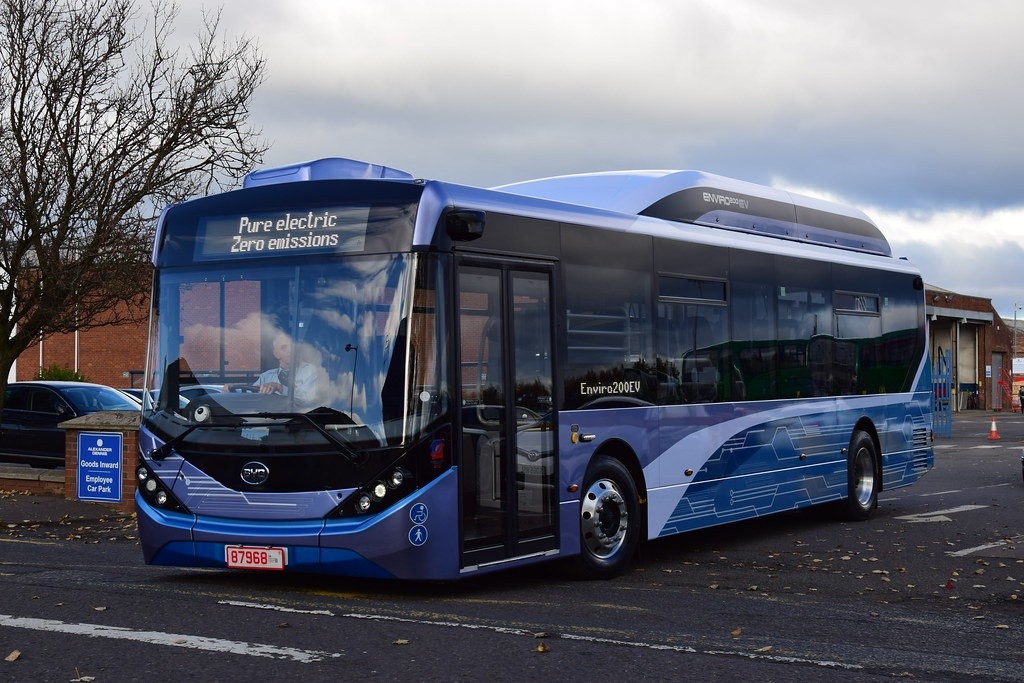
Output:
[{"left": 986, "top": 417, "right": 1001, "bottom": 440}]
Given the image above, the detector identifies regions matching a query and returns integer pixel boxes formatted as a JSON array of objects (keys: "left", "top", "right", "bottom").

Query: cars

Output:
[
  {"left": 0, "top": 381, "right": 142, "bottom": 469},
  {"left": 59, "top": 382, "right": 249, "bottom": 411}
]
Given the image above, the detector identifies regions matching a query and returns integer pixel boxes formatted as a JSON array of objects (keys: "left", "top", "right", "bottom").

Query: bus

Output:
[{"left": 129, "top": 154, "right": 936, "bottom": 583}]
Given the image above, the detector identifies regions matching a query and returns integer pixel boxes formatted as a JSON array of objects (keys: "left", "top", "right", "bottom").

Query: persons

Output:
[
  {"left": 1018, "top": 386, "right": 1024, "bottom": 416},
  {"left": 223, "top": 328, "right": 321, "bottom": 405}
]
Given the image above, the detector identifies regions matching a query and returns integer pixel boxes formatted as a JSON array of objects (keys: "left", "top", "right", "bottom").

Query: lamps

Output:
[
  {"left": 945, "top": 295, "right": 953, "bottom": 303},
  {"left": 932, "top": 295, "right": 941, "bottom": 303}
]
